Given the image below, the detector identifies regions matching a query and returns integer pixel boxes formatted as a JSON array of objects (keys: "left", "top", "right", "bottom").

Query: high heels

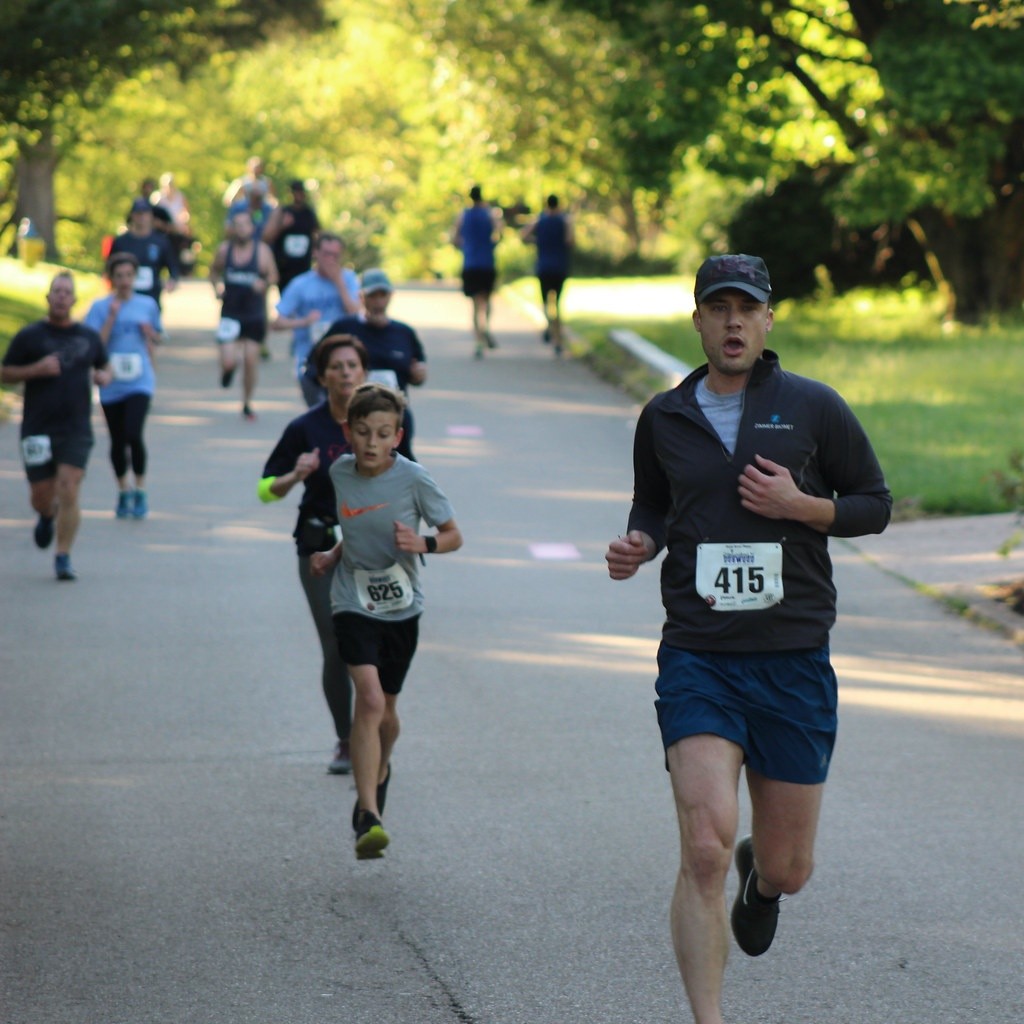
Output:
[{"left": 56, "top": 554, "right": 77, "bottom": 579}]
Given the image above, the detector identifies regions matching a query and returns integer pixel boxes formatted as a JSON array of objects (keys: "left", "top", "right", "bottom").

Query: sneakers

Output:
[
  {"left": 355, "top": 812, "right": 391, "bottom": 860},
  {"left": 731, "top": 834, "right": 782, "bottom": 957},
  {"left": 353, "top": 763, "right": 391, "bottom": 834}
]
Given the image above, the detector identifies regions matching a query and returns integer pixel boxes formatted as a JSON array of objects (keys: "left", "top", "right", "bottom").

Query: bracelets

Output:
[{"left": 264, "top": 277, "right": 271, "bottom": 288}]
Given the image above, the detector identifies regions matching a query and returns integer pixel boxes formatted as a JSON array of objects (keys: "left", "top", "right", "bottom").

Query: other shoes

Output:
[
  {"left": 35, "top": 504, "right": 58, "bottom": 548},
  {"left": 117, "top": 488, "right": 148, "bottom": 519},
  {"left": 328, "top": 754, "right": 353, "bottom": 772}
]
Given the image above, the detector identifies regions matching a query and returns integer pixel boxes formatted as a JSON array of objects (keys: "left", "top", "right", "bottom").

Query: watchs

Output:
[{"left": 422, "top": 534, "right": 438, "bottom": 554}]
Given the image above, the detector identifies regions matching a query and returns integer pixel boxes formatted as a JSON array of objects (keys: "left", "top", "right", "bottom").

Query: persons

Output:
[
  {"left": 449, "top": 186, "right": 503, "bottom": 360},
  {"left": 257, "top": 335, "right": 418, "bottom": 775},
  {"left": 0, "top": 269, "right": 113, "bottom": 582},
  {"left": 606, "top": 254, "right": 893, "bottom": 1024},
  {"left": 309, "top": 383, "right": 463, "bottom": 860},
  {"left": 520, "top": 195, "right": 574, "bottom": 354},
  {"left": 104, "top": 170, "right": 193, "bottom": 369},
  {"left": 82, "top": 254, "right": 165, "bottom": 520},
  {"left": 209, "top": 156, "right": 427, "bottom": 421}
]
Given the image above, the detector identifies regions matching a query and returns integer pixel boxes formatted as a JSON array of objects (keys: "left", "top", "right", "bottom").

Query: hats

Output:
[
  {"left": 694, "top": 254, "right": 772, "bottom": 305},
  {"left": 360, "top": 270, "right": 392, "bottom": 297}
]
